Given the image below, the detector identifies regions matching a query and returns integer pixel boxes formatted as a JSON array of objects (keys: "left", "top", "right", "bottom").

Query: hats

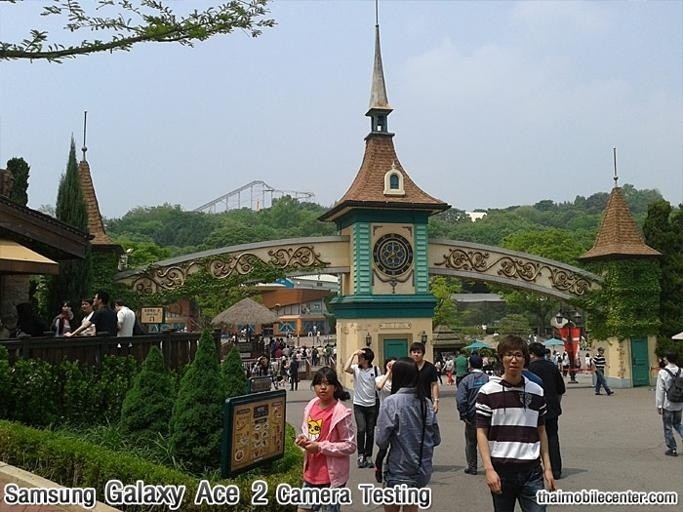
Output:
[
  {"left": 598, "top": 347, "right": 604, "bottom": 351},
  {"left": 292, "top": 355, "right": 298, "bottom": 359}
]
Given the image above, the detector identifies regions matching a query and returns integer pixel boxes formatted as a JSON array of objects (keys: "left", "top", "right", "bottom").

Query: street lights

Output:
[{"left": 556, "top": 303, "right": 582, "bottom": 384}]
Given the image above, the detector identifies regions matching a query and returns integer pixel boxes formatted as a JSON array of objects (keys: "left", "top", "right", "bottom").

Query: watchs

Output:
[{"left": 433, "top": 398, "right": 439, "bottom": 401}]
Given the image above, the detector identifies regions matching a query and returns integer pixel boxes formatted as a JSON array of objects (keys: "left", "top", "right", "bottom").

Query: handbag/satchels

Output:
[{"left": 374, "top": 366, "right": 380, "bottom": 416}]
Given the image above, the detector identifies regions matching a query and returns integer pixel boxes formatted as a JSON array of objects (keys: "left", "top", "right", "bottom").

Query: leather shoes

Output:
[
  {"left": 464, "top": 468, "right": 478, "bottom": 475},
  {"left": 553, "top": 475, "right": 561, "bottom": 480},
  {"left": 595, "top": 393, "right": 602, "bottom": 395},
  {"left": 608, "top": 391, "right": 613, "bottom": 395}
]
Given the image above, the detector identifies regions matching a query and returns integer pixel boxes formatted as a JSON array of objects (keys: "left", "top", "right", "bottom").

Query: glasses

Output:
[
  {"left": 502, "top": 351, "right": 526, "bottom": 362},
  {"left": 358, "top": 356, "right": 366, "bottom": 359}
]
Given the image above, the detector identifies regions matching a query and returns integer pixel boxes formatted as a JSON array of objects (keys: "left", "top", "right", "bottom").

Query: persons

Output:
[
  {"left": 80, "top": 298, "right": 96, "bottom": 338},
  {"left": 375, "top": 357, "right": 397, "bottom": 483},
  {"left": 409, "top": 342, "right": 439, "bottom": 414},
  {"left": 591, "top": 347, "right": 614, "bottom": 395},
  {"left": 441, "top": 355, "right": 455, "bottom": 385},
  {"left": 453, "top": 351, "right": 470, "bottom": 388},
  {"left": 10, "top": 303, "right": 36, "bottom": 337},
  {"left": 434, "top": 357, "right": 443, "bottom": 385},
  {"left": 227, "top": 327, "right": 337, "bottom": 391},
  {"left": 527, "top": 342, "right": 566, "bottom": 479},
  {"left": 456, "top": 354, "right": 489, "bottom": 475},
  {"left": 475, "top": 335, "right": 557, "bottom": 512},
  {"left": 49, "top": 301, "right": 74, "bottom": 338},
  {"left": 344, "top": 348, "right": 381, "bottom": 468},
  {"left": 545, "top": 349, "right": 593, "bottom": 377},
  {"left": 111, "top": 299, "right": 135, "bottom": 337},
  {"left": 480, "top": 355, "right": 489, "bottom": 373},
  {"left": 63, "top": 290, "right": 118, "bottom": 337},
  {"left": 295, "top": 366, "right": 357, "bottom": 512},
  {"left": 656, "top": 350, "right": 683, "bottom": 457},
  {"left": 376, "top": 357, "right": 441, "bottom": 512},
  {"left": 521, "top": 350, "right": 544, "bottom": 388}
]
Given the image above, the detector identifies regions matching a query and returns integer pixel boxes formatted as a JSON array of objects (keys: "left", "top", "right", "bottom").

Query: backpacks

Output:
[
  {"left": 663, "top": 367, "right": 683, "bottom": 403},
  {"left": 558, "top": 357, "right": 562, "bottom": 362}
]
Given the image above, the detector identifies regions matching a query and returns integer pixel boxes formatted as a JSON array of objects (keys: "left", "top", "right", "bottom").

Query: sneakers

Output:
[
  {"left": 665, "top": 449, "right": 677, "bottom": 456},
  {"left": 365, "top": 456, "right": 374, "bottom": 468},
  {"left": 357, "top": 453, "right": 365, "bottom": 468}
]
